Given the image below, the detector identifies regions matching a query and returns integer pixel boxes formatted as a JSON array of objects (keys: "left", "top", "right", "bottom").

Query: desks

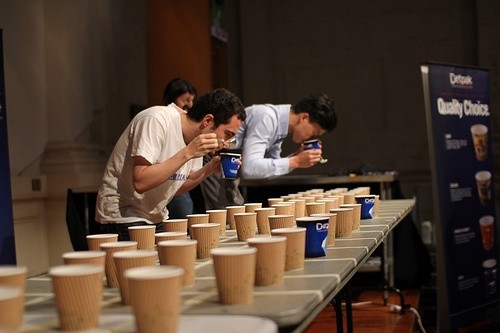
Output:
[
  {"left": 239, "top": 172, "right": 400, "bottom": 306},
  {"left": 1, "top": 198, "right": 415, "bottom": 333}
]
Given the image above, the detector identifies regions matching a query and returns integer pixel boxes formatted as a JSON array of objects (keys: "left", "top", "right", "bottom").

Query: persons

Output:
[
  {"left": 161, "top": 78, "right": 198, "bottom": 222},
  {"left": 95, "top": 87, "right": 247, "bottom": 242},
  {"left": 200, "top": 93, "right": 337, "bottom": 225}
]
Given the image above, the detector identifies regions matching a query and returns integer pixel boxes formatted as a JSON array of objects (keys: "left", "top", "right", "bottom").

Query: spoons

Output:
[{"left": 319, "top": 155, "right": 328, "bottom": 163}]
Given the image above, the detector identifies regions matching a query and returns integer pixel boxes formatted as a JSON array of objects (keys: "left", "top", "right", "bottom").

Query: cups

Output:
[
  {"left": 220, "top": 153, "right": 241, "bottom": 179},
  {"left": 0, "top": 286, "right": 23, "bottom": 331},
  {"left": 0, "top": 265, "right": 27, "bottom": 306},
  {"left": 113, "top": 250, "right": 157, "bottom": 306},
  {"left": 128, "top": 225, "right": 156, "bottom": 250},
  {"left": 60, "top": 251, "right": 107, "bottom": 269},
  {"left": 483, "top": 258, "right": 497, "bottom": 288},
  {"left": 86, "top": 234, "right": 118, "bottom": 251},
  {"left": 305, "top": 139, "right": 320, "bottom": 151},
  {"left": 475, "top": 170, "right": 492, "bottom": 200},
  {"left": 124, "top": 266, "right": 184, "bottom": 333},
  {"left": 99, "top": 241, "right": 137, "bottom": 289},
  {"left": 48, "top": 264, "right": 104, "bottom": 330},
  {"left": 155, "top": 187, "right": 380, "bottom": 301},
  {"left": 481, "top": 216, "right": 494, "bottom": 249},
  {"left": 471, "top": 123, "right": 487, "bottom": 154}
]
